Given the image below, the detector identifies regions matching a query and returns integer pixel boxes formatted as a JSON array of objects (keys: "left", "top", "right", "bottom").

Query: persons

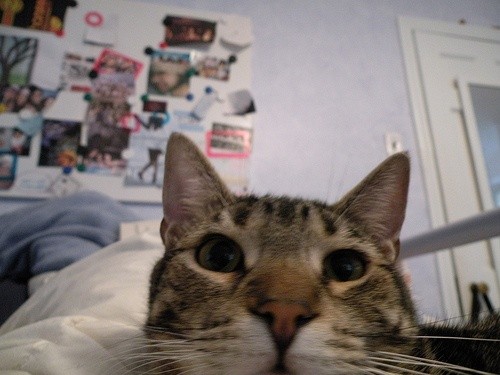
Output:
[{"left": 0, "top": 0, "right": 218, "bottom": 188}]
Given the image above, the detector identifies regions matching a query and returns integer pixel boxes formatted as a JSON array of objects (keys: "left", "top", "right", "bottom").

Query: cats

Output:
[{"left": 103, "top": 132, "right": 499, "bottom": 375}]
